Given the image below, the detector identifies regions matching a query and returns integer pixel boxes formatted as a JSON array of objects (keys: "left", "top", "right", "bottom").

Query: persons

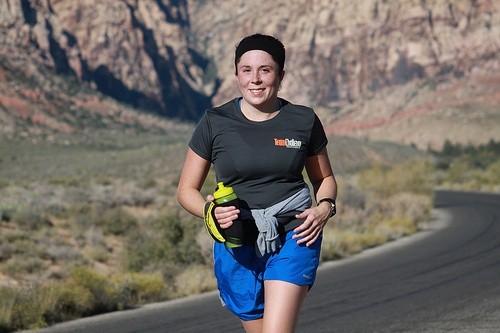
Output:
[{"left": 176, "top": 32, "right": 338, "bottom": 333}]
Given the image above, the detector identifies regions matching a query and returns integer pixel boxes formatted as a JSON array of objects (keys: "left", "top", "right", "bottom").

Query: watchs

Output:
[{"left": 315, "top": 198, "right": 337, "bottom": 218}]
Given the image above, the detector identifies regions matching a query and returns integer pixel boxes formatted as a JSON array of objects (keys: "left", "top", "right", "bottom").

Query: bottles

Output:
[{"left": 212, "top": 181, "right": 247, "bottom": 247}]
